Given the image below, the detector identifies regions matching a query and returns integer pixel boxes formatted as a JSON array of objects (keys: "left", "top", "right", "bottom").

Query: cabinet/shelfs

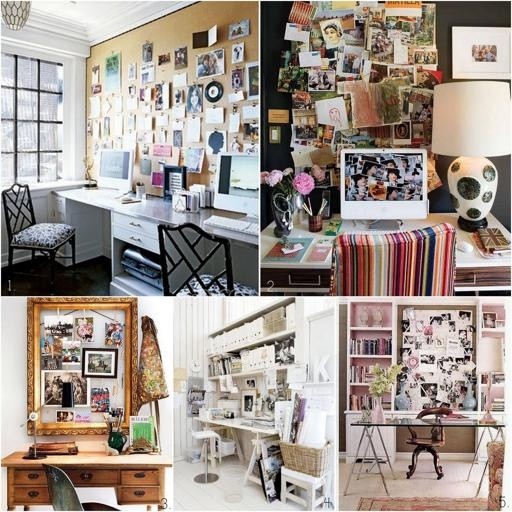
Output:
[
  {"left": 203, "top": 297, "right": 310, "bottom": 384},
  {"left": 471, "top": 410, "right": 504, "bottom": 466},
  {"left": 344, "top": 298, "right": 399, "bottom": 413},
  {"left": 474, "top": 297, "right": 504, "bottom": 415},
  {"left": 343, "top": 410, "right": 398, "bottom": 466}
]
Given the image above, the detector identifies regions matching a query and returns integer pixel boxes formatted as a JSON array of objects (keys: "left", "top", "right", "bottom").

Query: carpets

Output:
[{"left": 355, "top": 494, "right": 490, "bottom": 510}]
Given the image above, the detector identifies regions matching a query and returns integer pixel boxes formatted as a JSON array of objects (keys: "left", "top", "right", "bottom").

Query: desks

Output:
[
  {"left": 342, "top": 416, "right": 504, "bottom": 498},
  {"left": 260, "top": 205, "right": 512, "bottom": 296},
  {"left": 1, "top": 445, "right": 172, "bottom": 511},
  {"left": 343, "top": 408, "right": 505, "bottom": 465},
  {"left": 190, "top": 412, "right": 299, "bottom": 491},
  {"left": 44, "top": 186, "right": 262, "bottom": 298}
]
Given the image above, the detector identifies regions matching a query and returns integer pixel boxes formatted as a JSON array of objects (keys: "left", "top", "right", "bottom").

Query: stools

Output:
[{"left": 278, "top": 462, "right": 332, "bottom": 511}]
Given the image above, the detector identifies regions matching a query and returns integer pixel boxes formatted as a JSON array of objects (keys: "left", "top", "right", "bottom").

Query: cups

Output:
[
  {"left": 135, "top": 185, "right": 146, "bottom": 201},
  {"left": 108, "top": 431, "right": 127, "bottom": 453},
  {"left": 308, "top": 215, "right": 323, "bottom": 233}
]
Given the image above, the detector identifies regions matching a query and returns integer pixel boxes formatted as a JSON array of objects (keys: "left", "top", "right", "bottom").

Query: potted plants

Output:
[{"left": 366, "top": 361, "right": 401, "bottom": 427}]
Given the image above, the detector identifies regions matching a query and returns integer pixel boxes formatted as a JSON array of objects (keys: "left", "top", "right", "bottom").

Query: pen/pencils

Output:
[{"left": 301, "top": 197, "right": 328, "bottom": 216}]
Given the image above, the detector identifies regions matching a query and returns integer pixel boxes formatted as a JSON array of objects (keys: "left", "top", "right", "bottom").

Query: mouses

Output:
[
  {"left": 120, "top": 196, "right": 133, "bottom": 201},
  {"left": 456, "top": 241, "right": 474, "bottom": 254}
]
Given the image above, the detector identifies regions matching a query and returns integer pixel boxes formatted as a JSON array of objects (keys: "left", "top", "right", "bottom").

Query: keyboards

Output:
[{"left": 203, "top": 214, "right": 259, "bottom": 237}]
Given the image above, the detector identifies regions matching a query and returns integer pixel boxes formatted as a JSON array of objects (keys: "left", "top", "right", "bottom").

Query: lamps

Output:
[
  {"left": 226, "top": 378, "right": 241, "bottom": 400},
  {"left": 473, "top": 336, "right": 504, "bottom": 426},
  {"left": 1, "top": 1, "right": 34, "bottom": 31},
  {"left": 429, "top": 79, "right": 512, "bottom": 234},
  {"left": 18, "top": 380, "right": 78, "bottom": 461}
]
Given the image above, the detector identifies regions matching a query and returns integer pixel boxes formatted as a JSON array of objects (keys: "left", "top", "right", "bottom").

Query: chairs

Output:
[
  {"left": 155, "top": 221, "right": 259, "bottom": 298},
  {"left": 2, "top": 181, "right": 79, "bottom": 296},
  {"left": 403, "top": 405, "right": 453, "bottom": 480},
  {"left": 324, "top": 222, "right": 458, "bottom": 297},
  {"left": 40, "top": 462, "right": 122, "bottom": 511},
  {"left": 183, "top": 418, "right": 223, "bottom": 485}
]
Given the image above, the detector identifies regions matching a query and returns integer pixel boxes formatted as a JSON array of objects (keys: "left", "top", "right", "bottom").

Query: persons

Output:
[
  {"left": 233, "top": 46, "right": 242, "bottom": 59},
  {"left": 197, "top": 53, "right": 223, "bottom": 77},
  {"left": 472, "top": 47, "right": 496, "bottom": 62},
  {"left": 186, "top": 85, "right": 202, "bottom": 112},
  {"left": 42, "top": 319, "right": 124, "bottom": 422},
  {"left": 245, "top": 397, "right": 253, "bottom": 412},
  {"left": 401, "top": 312, "right": 496, "bottom": 407},
  {"left": 176, "top": 49, "right": 186, "bottom": 65},
  {"left": 277, "top": 22, "right": 437, "bottom": 201}
]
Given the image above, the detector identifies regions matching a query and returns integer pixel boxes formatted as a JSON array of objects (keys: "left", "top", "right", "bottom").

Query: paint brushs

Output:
[{"left": 103, "top": 407, "right": 123, "bottom": 432}]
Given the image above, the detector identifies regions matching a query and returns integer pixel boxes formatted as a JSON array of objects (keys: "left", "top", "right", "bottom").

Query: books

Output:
[
  {"left": 349, "top": 337, "right": 392, "bottom": 411},
  {"left": 209, "top": 351, "right": 241, "bottom": 376},
  {"left": 174, "top": 182, "right": 214, "bottom": 212},
  {"left": 261, "top": 237, "right": 316, "bottom": 265},
  {"left": 128, "top": 415, "right": 157, "bottom": 448},
  {"left": 305, "top": 245, "right": 332, "bottom": 264},
  {"left": 481, "top": 370, "right": 505, "bottom": 411},
  {"left": 477, "top": 227, "right": 511, "bottom": 254}
]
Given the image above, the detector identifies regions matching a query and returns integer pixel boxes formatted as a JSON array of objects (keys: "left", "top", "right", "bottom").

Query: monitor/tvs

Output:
[
  {"left": 97, "top": 149, "right": 134, "bottom": 196},
  {"left": 214, "top": 152, "right": 260, "bottom": 222},
  {"left": 340, "top": 148, "right": 427, "bottom": 231}
]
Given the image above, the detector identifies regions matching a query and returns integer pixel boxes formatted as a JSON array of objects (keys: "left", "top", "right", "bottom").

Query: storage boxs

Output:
[{"left": 206, "top": 302, "right": 296, "bottom": 374}]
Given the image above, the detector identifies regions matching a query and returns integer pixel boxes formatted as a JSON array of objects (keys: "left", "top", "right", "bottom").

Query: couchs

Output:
[{"left": 484, "top": 437, "right": 503, "bottom": 510}]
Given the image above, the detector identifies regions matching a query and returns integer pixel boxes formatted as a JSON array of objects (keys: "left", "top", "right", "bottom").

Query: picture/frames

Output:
[
  {"left": 38, "top": 367, "right": 93, "bottom": 408},
  {"left": 26, "top": 295, "right": 140, "bottom": 439},
  {"left": 448, "top": 23, "right": 510, "bottom": 82},
  {"left": 238, "top": 388, "right": 259, "bottom": 418},
  {"left": 80, "top": 346, "right": 119, "bottom": 380}
]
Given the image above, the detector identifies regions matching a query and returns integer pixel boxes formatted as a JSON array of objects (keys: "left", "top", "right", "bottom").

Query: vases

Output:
[
  {"left": 279, "top": 192, "right": 303, "bottom": 250},
  {"left": 105, "top": 428, "right": 129, "bottom": 455}
]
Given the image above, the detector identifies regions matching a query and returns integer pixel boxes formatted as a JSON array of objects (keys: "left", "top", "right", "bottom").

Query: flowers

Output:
[{"left": 258, "top": 164, "right": 329, "bottom": 209}]
